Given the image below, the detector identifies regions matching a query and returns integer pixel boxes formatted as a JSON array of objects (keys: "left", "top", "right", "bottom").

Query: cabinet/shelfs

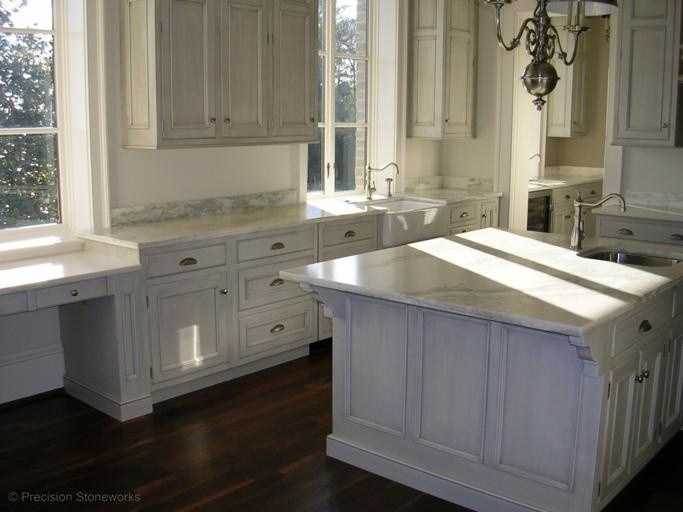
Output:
[
  {"left": 0, "top": 277, "right": 110, "bottom": 314},
  {"left": 272, "top": 0, "right": 319, "bottom": 144},
  {"left": 234, "top": 213, "right": 379, "bottom": 367},
  {"left": 550, "top": 182, "right": 602, "bottom": 236},
  {"left": 595, "top": 214, "right": 682, "bottom": 248},
  {"left": 448, "top": 197, "right": 500, "bottom": 236},
  {"left": 135, "top": 238, "right": 233, "bottom": 409},
  {"left": 118, "top": 1, "right": 272, "bottom": 151},
  {"left": 548, "top": 15, "right": 599, "bottom": 139},
  {"left": 592, "top": 280, "right": 683, "bottom": 512},
  {"left": 404, "top": 1, "right": 481, "bottom": 140},
  {"left": 607, "top": 0, "right": 682, "bottom": 148}
]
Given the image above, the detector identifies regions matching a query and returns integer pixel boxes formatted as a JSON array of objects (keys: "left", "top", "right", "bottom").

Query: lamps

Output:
[{"left": 482, "top": 0, "right": 593, "bottom": 110}]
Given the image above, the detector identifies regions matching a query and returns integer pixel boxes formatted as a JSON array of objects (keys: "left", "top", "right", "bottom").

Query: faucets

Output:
[
  {"left": 571, "top": 191, "right": 626, "bottom": 248},
  {"left": 367, "top": 162, "right": 399, "bottom": 199},
  {"left": 529, "top": 153, "right": 542, "bottom": 180}
]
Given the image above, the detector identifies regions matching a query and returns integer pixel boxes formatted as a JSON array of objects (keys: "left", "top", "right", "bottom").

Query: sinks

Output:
[
  {"left": 363, "top": 199, "right": 450, "bottom": 248},
  {"left": 577, "top": 246, "right": 683, "bottom": 268}
]
